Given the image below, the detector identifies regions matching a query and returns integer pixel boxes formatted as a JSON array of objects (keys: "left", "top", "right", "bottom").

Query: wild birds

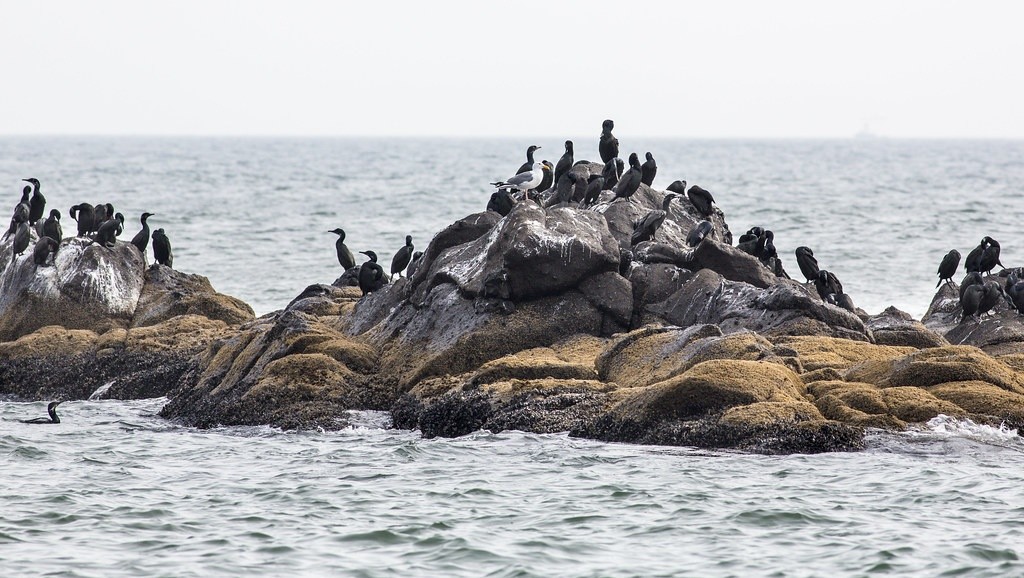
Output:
[
  {"left": 5, "top": 178, "right": 63, "bottom": 272},
  {"left": 631, "top": 181, "right": 843, "bottom": 297},
  {"left": 329, "top": 228, "right": 423, "bottom": 294},
  {"left": 69, "top": 203, "right": 174, "bottom": 267},
  {"left": 19, "top": 401, "right": 65, "bottom": 424},
  {"left": 489, "top": 120, "right": 658, "bottom": 206},
  {"left": 935, "top": 236, "right": 1024, "bottom": 321}
]
